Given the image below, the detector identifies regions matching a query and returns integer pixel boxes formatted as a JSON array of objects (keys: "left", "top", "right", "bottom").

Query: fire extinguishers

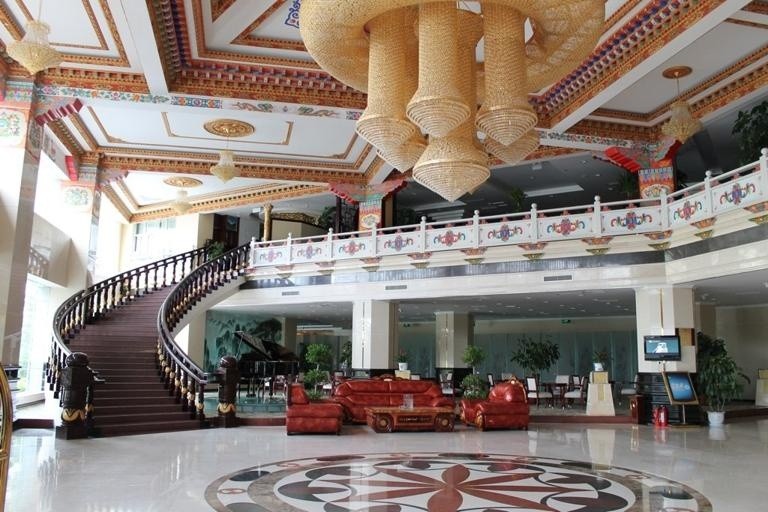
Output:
[{"left": 659, "top": 406, "right": 667, "bottom": 427}]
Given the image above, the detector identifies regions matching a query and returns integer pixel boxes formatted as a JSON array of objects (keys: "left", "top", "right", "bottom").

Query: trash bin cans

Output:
[{"left": 4, "top": 366, "right": 22, "bottom": 421}]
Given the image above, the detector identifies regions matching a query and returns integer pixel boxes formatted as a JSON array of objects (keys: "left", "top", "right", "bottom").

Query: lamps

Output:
[
  {"left": 659, "top": 66, "right": 703, "bottom": 145},
  {"left": 298, "top": 1, "right": 605, "bottom": 203},
  {"left": 164, "top": 176, "right": 202, "bottom": 217},
  {"left": 6, "top": 1, "right": 63, "bottom": 77},
  {"left": 204, "top": 119, "right": 254, "bottom": 185}
]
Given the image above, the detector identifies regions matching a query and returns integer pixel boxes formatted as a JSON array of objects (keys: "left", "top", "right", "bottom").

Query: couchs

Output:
[{"left": 330, "top": 378, "right": 457, "bottom": 425}]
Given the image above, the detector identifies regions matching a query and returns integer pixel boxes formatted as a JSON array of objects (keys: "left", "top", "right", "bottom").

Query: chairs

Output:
[
  {"left": 285, "top": 382, "right": 345, "bottom": 435},
  {"left": 487, "top": 370, "right": 641, "bottom": 412},
  {"left": 458, "top": 377, "right": 529, "bottom": 431}
]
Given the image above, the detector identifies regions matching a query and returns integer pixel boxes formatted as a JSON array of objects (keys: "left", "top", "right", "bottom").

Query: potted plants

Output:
[
  {"left": 592, "top": 349, "right": 615, "bottom": 372},
  {"left": 691, "top": 330, "right": 752, "bottom": 424},
  {"left": 394, "top": 352, "right": 411, "bottom": 370}
]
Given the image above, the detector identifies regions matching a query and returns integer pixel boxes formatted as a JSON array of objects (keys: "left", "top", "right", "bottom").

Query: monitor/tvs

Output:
[{"left": 644, "top": 336, "right": 681, "bottom": 360}]
